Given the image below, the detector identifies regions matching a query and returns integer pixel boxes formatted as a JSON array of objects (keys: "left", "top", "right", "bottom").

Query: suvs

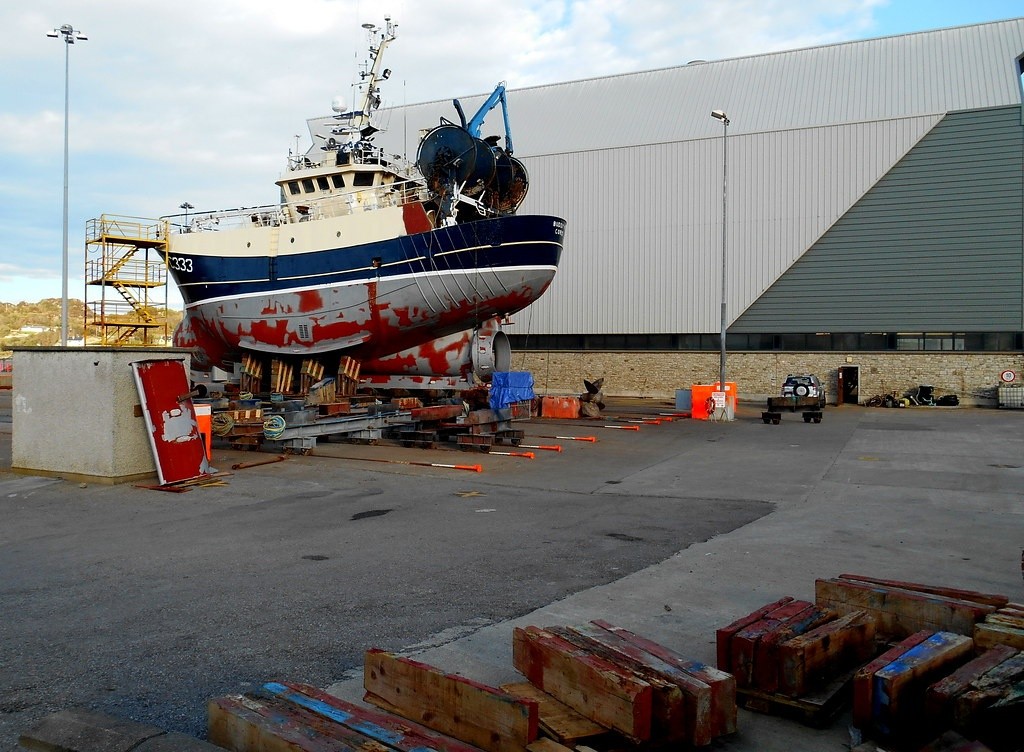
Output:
[{"left": 781, "top": 372, "right": 827, "bottom": 410}]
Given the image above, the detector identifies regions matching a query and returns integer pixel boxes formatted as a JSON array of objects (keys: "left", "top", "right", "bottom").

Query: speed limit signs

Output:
[{"left": 1002, "top": 370, "right": 1015, "bottom": 382}]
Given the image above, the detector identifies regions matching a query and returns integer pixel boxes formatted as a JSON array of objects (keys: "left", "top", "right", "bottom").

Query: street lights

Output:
[
  {"left": 47, "top": 24, "right": 90, "bottom": 347},
  {"left": 710, "top": 109, "right": 731, "bottom": 397}
]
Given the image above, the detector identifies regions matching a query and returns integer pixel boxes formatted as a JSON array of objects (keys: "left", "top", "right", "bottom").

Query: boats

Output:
[{"left": 156, "top": 19, "right": 566, "bottom": 399}]
